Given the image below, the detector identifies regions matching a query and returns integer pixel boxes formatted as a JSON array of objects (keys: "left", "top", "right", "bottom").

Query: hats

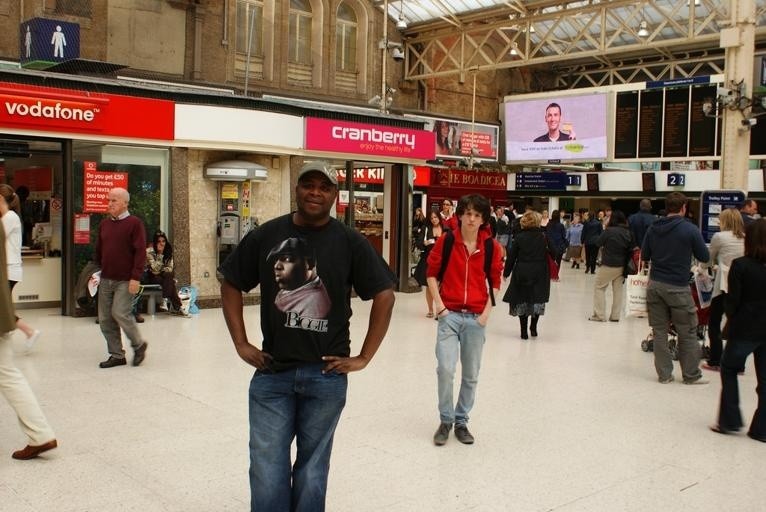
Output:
[{"left": 297, "top": 161, "right": 338, "bottom": 190}]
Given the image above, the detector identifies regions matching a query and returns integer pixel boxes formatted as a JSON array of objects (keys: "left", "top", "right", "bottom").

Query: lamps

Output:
[
  {"left": 509, "top": 7, "right": 536, "bottom": 58},
  {"left": 396, "top": 0, "right": 408, "bottom": 33},
  {"left": 687, "top": 0, "right": 703, "bottom": 7},
  {"left": 636, "top": 3, "right": 650, "bottom": 38}
]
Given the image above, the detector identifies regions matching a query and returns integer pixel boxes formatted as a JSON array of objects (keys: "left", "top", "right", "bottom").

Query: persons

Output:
[
  {"left": 424, "top": 196, "right": 503, "bottom": 444},
  {"left": 266, "top": 238, "right": 330, "bottom": 324},
  {"left": 93, "top": 187, "right": 148, "bottom": 368},
  {"left": 431, "top": 118, "right": 457, "bottom": 156},
  {"left": 96, "top": 283, "right": 144, "bottom": 323},
  {"left": 15, "top": 185, "right": 40, "bottom": 247},
  {"left": 218, "top": 160, "right": 396, "bottom": 511},
  {"left": 413, "top": 195, "right": 658, "bottom": 339},
  {"left": 710, "top": 218, "right": 766, "bottom": 443},
  {"left": 142, "top": 230, "right": 188, "bottom": 316},
  {"left": 0, "top": 182, "right": 39, "bottom": 352},
  {"left": 534, "top": 103, "right": 575, "bottom": 141},
  {"left": 640, "top": 191, "right": 757, "bottom": 386},
  {"left": 0, "top": 221, "right": 57, "bottom": 460}
]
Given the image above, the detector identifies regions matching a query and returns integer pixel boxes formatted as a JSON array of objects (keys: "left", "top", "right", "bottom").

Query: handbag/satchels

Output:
[
  {"left": 625, "top": 255, "right": 638, "bottom": 275},
  {"left": 548, "top": 254, "right": 558, "bottom": 280}
]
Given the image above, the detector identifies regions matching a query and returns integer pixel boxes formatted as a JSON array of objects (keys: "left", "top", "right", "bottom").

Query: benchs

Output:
[{"left": 86, "top": 278, "right": 177, "bottom": 313}]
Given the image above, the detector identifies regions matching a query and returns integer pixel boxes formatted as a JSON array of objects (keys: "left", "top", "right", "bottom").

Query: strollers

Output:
[{"left": 638, "top": 264, "right": 713, "bottom": 364}]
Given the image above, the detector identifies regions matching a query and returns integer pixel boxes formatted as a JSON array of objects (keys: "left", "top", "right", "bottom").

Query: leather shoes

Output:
[
  {"left": 100, "top": 343, "right": 147, "bottom": 368},
  {"left": 434, "top": 423, "right": 473, "bottom": 445},
  {"left": 12, "top": 440, "right": 56, "bottom": 460}
]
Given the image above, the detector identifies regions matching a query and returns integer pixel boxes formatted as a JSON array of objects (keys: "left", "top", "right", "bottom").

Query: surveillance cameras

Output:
[
  {"left": 392, "top": 48, "right": 405, "bottom": 61},
  {"left": 718, "top": 87, "right": 733, "bottom": 96},
  {"left": 703, "top": 103, "right": 712, "bottom": 112},
  {"left": 741, "top": 118, "right": 757, "bottom": 126}
]
